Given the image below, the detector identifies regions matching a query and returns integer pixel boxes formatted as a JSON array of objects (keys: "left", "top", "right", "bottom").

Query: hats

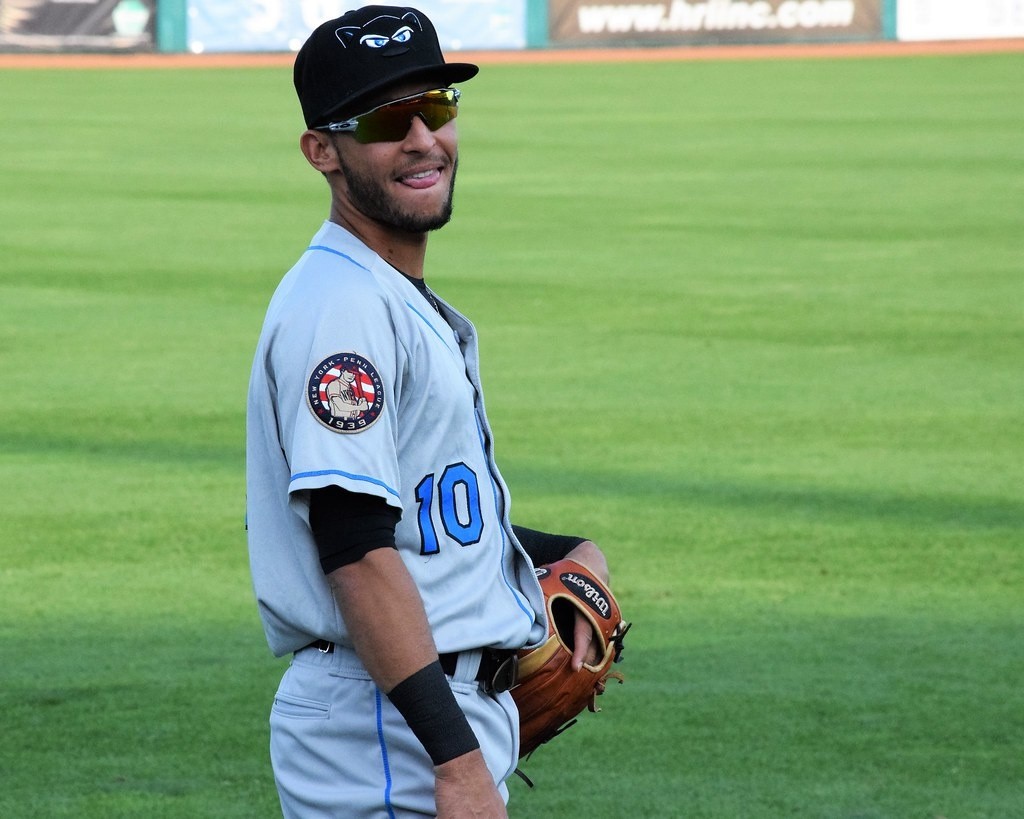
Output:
[{"left": 294, "top": 5, "right": 480, "bottom": 130}]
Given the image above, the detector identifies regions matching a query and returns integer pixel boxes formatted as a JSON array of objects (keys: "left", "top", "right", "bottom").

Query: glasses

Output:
[{"left": 313, "top": 87, "right": 462, "bottom": 145}]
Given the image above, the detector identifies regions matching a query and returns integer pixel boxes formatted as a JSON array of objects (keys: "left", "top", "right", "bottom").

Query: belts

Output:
[{"left": 306, "top": 639, "right": 520, "bottom": 695}]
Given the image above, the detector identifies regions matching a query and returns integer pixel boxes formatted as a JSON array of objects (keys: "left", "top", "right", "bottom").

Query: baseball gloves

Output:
[{"left": 502, "top": 556, "right": 629, "bottom": 760}]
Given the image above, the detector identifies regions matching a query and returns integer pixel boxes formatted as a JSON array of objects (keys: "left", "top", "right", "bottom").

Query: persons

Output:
[{"left": 246, "top": 5, "right": 611, "bottom": 819}]
